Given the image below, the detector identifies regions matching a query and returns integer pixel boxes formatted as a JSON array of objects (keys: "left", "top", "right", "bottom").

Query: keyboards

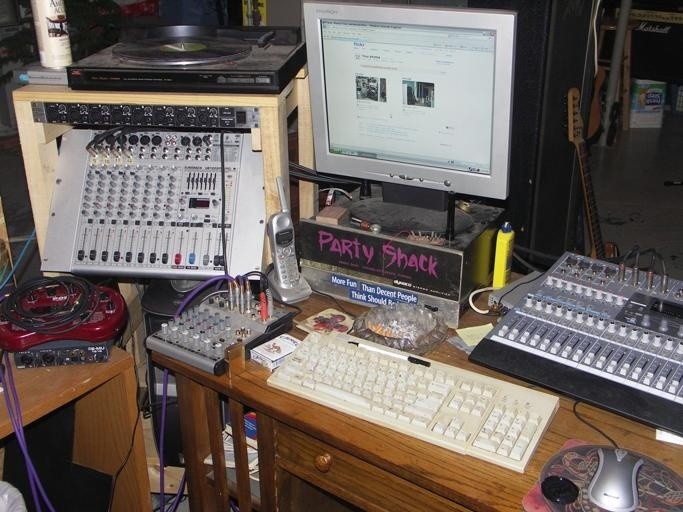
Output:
[{"left": 266, "top": 329, "right": 561, "bottom": 473}]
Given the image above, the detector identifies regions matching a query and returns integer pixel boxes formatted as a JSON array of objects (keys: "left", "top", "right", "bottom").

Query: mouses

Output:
[{"left": 588, "top": 447, "right": 644, "bottom": 512}]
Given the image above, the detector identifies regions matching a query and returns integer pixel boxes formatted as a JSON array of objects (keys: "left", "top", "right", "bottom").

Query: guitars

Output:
[{"left": 562, "top": 87, "right": 619, "bottom": 260}]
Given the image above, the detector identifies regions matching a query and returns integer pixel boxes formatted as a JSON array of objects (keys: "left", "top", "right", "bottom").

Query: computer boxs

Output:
[{"left": 144, "top": 312, "right": 226, "bottom": 468}]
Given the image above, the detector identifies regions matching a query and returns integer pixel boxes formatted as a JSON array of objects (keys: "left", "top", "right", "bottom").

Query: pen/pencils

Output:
[{"left": 348, "top": 341, "right": 431, "bottom": 367}]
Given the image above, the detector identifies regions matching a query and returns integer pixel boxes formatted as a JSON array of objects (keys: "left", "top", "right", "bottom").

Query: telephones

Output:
[{"left": 265, "top": 176, "right": 312, "bottom": 305}]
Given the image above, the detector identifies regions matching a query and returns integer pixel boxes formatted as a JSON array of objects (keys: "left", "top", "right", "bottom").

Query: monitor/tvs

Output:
[{"left": 303, "top": 1, "right": 517, "bottom": 201}]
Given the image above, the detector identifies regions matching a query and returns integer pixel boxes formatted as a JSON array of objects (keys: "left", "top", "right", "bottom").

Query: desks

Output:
[
  {"left": 0, "top": 308, "right": 150, "bottom": 510},
  {"left": 149, "top": 270, "right": 683, "bottom": 510}
]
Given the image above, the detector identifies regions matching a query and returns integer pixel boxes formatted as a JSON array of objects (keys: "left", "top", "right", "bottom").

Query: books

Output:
[
  {"left": 202, "top": 447, "right": 258, "bottom": 471},
  {"left": 248, "top": 471, "right": 261, "bottom": 483},
  {"left": 247, "top": 469, "right": 259, "bottom": 475},
  {"left": 292, "top": 306, "right": 362, "bottom": 335},
  {"left": 222, "top": 409, "right": 256, "bottom": 451},
  {"left": 220, "top": 430, "right": 257, "bottom": 454}
]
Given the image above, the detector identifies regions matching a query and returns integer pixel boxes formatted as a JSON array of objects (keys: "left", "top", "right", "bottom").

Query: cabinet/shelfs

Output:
[{"left": 11, "top": 62, "right": 319, "bottom": 497}]
